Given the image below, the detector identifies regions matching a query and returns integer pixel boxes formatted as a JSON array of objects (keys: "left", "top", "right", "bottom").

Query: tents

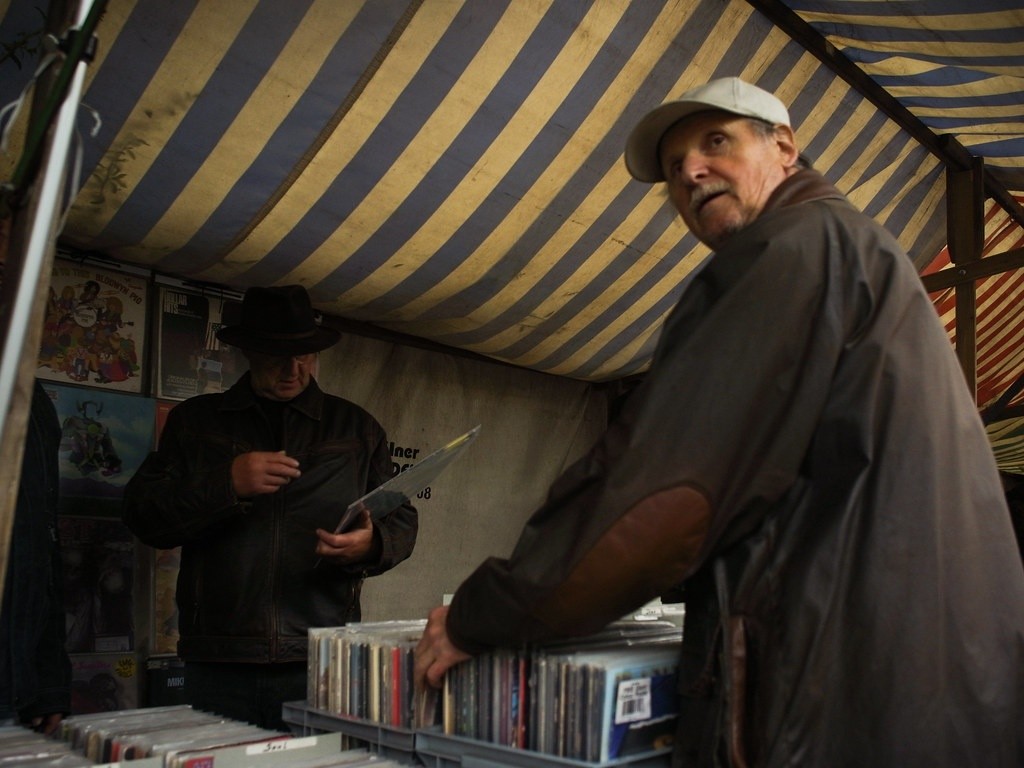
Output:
[{"left": 0, "top": 2, "right": 1022, "bottom": 488}]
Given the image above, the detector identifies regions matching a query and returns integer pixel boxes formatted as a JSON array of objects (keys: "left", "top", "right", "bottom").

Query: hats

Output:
[
  {"left": 625, "top": 76, "right": 791, "bottom": 181},
  {"left": 215, "top": 285, "right": 341, "bottom": 358}
]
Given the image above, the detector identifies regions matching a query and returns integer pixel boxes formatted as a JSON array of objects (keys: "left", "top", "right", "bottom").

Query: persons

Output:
[
  {"left": 127, "top": 284, "right": 420, "bottom": 731},
  {"left": 410, "top": 78, "right": 1024, "bottom": 768}
]
